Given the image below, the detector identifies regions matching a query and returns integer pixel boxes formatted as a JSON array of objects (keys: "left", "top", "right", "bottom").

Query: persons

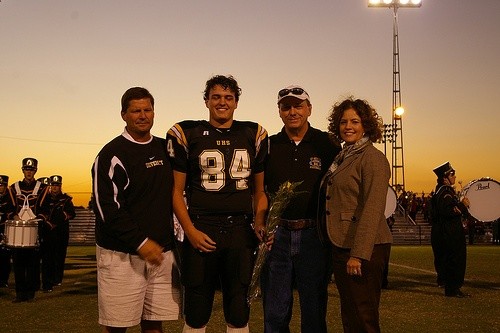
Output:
[
  {"left": 316, "top": 100, "right": 392, "bottom": 333},
  {"left": 90, "top": 86, "right": 182, "bottom": 333},
  {"left": 399, "top": 191, "right": 433, "bottom": 221},
  {"left": 467, "top": 215, "right": 500, "bottom": 244},
  {"left": 262, "top": 86, "right": 342, "bottom": 333},
  {"left": 424, "top": 162, "right": 472, "bottom": 298},
  {"left": 167, "top": 75, "right": 273, "bottom": 333},
  {"left": 0, "top": 158, "right": 76, "bottom": 303}
]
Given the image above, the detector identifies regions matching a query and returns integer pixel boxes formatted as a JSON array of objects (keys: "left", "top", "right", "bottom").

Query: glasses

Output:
[{"left": 278, "top": 88, "right": 309, "bottom": 98}]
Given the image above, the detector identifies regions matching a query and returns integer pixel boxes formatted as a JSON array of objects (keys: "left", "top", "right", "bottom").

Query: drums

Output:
[
  {"left": 459, "top": 177, "right": 500, "bottom": 226},
  {"left": 383, "top": 185, "right": 397, "bottom": 220},
  {"left": 3, "top": 219, "right": 40, "bottom": 249}
]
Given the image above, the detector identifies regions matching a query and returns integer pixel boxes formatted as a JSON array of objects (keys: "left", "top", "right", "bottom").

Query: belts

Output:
[{"left": 277, "top": 219, "right": 319, "bottom": 230}]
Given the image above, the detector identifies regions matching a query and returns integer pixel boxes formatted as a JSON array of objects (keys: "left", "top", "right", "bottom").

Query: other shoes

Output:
[
  {"left": 445, "top": 289, "right": 471, "bottom": 298},
  {"left": 26, "top": 298, "right": 33, "bottom": 303},
  {"left": 13, "top": 298, "right": 20, "bottom": 303}
]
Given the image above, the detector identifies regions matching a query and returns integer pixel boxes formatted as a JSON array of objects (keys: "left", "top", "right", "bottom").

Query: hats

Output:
[
  {"left": 37, "top": 177, "right": 50, "bottom": 186},
  {"left": 278, "top": 85, "right": 310, "bottom": 105},
  {"left": 22, "top": 158, "right": 37, "bottom": 171},
  {"left": 433, "top": 162, "right": 453, "bottom": 178},
  {"left": 50, "top": 175, "right": 62, "bottom": 186},
  {"left": 0, "top": 175, "right": 8, "bottom": 186}
]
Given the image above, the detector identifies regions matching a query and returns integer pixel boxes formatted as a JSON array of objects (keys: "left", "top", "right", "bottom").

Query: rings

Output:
[{"left": 353, "top": 272, "right": 357, "bottom": 274}]
